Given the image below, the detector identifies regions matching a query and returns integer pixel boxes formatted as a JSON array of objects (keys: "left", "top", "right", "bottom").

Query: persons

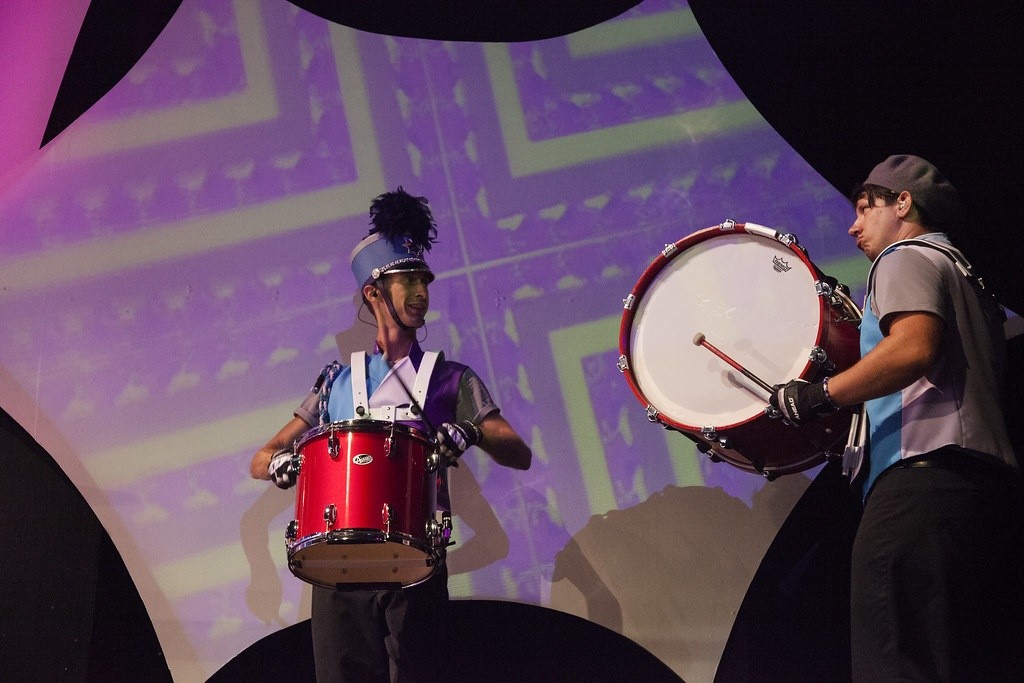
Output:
[
  {"left": 251, "top": 231, "right": 532, "bottom": 683},
  {"left": 769, "top": 156, "right": 1016, "bottom": 683}
]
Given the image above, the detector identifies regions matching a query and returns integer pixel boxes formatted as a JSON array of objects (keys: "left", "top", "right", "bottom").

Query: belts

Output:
[{"left": 893, "top": 452, "right": 1023, "bottom": 482}]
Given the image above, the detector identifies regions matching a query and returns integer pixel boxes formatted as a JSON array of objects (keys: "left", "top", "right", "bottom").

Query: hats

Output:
[
  {"left": 861, "top": 154, "right": 962, "bottom": 222},
  {"left": 349, "top": 185, "right": 440, "bottom": 332}
]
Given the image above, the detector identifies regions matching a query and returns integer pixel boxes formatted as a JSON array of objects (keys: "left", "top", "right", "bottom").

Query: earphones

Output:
[
  {"left": 370, "top": 291, "right": 378, "bottom": 298},
  {"left": 899, "top": 201, "right": 906, "bottom": 211}
]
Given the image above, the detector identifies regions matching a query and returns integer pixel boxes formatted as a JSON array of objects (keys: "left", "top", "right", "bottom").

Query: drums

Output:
[
  {"left": 620, "top": 220, "right": 864, "bottom": 479},
  {"left": 285, "top": 420, "right": 444, "bottom": 586}
]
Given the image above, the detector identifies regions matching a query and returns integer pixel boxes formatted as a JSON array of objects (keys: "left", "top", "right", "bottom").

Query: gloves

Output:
[
  {"left": 436, "top": 419, "right": 483, "bottom": 467},
  {"left": 769, "top": 376, "right": 842, "bottom": 429},
  {"left": 267, "top": 448, "right": 296, "bottom": 489}
]
